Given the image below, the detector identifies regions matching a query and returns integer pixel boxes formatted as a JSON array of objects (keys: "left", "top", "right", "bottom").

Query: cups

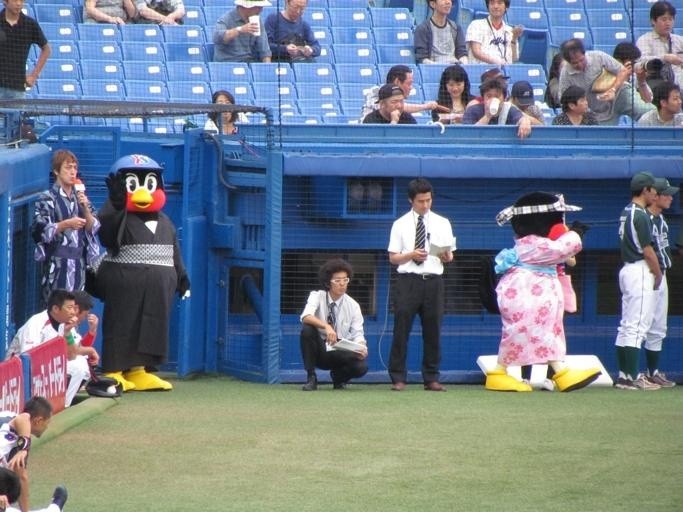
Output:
[
  {"left": 488, "top": 97, "right": 500, "bottom": 116},
  {"left": 247, "top": 15, "right": 260, "bottom": 37}
]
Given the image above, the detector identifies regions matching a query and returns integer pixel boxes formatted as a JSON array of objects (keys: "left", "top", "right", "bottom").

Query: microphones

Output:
[{"left": 73, "top": 178, "right": 85, "bottom": 207}]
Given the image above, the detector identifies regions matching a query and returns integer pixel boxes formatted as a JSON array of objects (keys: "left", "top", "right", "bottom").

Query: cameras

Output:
[{"left": 631, "top": 58, "right": 662, "bottom": 74}]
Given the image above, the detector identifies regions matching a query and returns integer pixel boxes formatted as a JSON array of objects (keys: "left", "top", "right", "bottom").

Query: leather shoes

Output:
[
  {"left": 53, "top": 486, "right": 68, "bottom": 510},
  {"left": 303, "top": 380, "right": 318, "bottom": 391},
  {"left": 392, "top": 382, "right": 404, "bottom": 390},
  {"left": 423, "top": 381, "right": 447, "bottom": 391},
  {"left": 333, "top": 382, "right": 347, "bottom": 389}
]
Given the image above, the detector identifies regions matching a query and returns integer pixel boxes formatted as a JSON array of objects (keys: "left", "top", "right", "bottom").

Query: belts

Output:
[{"left": 409, "top": 272, "right": 439, "bottom": 280}]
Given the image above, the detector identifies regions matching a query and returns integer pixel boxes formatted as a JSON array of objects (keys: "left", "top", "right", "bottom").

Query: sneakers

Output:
[
  {"left": 624, "top": 376, "right": 659, "bottom": 391},
  {"left": 646, "top": 373, "right": 676, "bottom": 388},
  {"left": 616, "top": 377, "right": 625, "bottom": 388}
]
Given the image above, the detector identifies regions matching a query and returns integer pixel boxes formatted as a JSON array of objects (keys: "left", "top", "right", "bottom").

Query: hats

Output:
[
  {"left": 375, "top": 83, "right": 406, "bottom": 104},
  {"left": 630, "top": 172, "right": 660, "bottom": 192},
  {"left": 590, "top": 66, "right": 617, "bottom": 93},
  {"left": 655, "top": 178, "right": 678, "bottom": 195},
  {"left": 109, "top": 155, "right": 165, "bottom": 176},
  {"left": 235, "top": 0, "right": 272, "bottom": 8},
  {"left": 511, "top": 81, "right": 534, "bottom": 106},
  {"left": 482, "top": 68, "right": 510, "bottom": 81},
  {"left": 495, "top": 194, "right": 582, "bottom": 227}
]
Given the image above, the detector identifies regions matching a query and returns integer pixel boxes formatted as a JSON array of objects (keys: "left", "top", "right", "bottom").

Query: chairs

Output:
[{"left": 0, "top": 0, "right": 683, "bottom": 132}]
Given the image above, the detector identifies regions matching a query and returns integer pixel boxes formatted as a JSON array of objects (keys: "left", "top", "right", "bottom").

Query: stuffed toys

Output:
[
  {"left": 85, "top": 155, "right": 190, "bottom": 392},
  {"left": 485, "top": 193, "right": 600, "bottom": 392}
]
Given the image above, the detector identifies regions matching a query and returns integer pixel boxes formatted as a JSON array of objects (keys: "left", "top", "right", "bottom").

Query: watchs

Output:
[{"left": 611, "top": 88, "right": 618, "bottom": 91}]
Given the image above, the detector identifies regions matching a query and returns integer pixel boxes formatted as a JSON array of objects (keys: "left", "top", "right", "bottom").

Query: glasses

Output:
[{"left": 330, "top": 278, "right": 349, "bottom": 283}]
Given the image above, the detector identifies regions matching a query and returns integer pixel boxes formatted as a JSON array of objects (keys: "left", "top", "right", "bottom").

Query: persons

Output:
[
  {"left": 614, "top": 172, "right": 662, "bottom": 390},
  {"left": 635, "top": 1, "right": 683, "bottom": 110},
  {"left": 646, "top": 174, "right": 681, "bottom": 388},
  {"left": 0, "top": 396, "right": 52, "bottom": 512},
  {"left": 4, "top": 290, "right": 90, "bottom": 406},
  {"left": 32, "top": 150, "right": 108, "bottom": 391},
  {"left": 466, "top": 69, "right": 510, "bottom": 110},
  {"left": 414, "top": 0, "right": 468, "bottom": 65},
  {"left": 213, "top": 0, "right": 272, "bottom": 62},
  {"left": 387, "top": 179, "right": 453, "bottom": 390},
  {"left": 64, "top": 291, "right": 98, "bottom": 390},
  {"left": 613, "top": 43, "right": 654, "bottom": 104},
  {"left": 0, "top": 468, "right": 68, "bottom": 512},
  {"left": 552, "top": 86, "right": 600, "bottom": 126},
  {"left": 557, "top": 39, "right": 656, "bottom": 126},
  {"left": 265, "top": 0, "right": 321, "bottom": 62},
  {"left": 364, "top": 65, "right": 437, "bottom": 124},
  {"left": 203, "top": 91, "right": 249, "bottom": 134},
  {"left": 300, "top": 259, "right": 368, "bottom": 390},
  {"left": 545, "top": 54, "right": 562, "bottom": 111},
  {"left": 83, "top": 0, "right": 138, "bottom": 24},
  {"left": 133, "top": 0, "right": 185, "bottom": 26},
  {"left": 506, "top": 81, "right": 544, "bottom": 125},
  {"left": 363, "top": 83, "right": 417, "bottom": 124},
  {"left": 636, "top": 83, "right": 683, "bottom": 127},
  {"left": 432, "top": 66, "right": 478, "bottom": 124},
  {"left": 461, "top": 78, "right": 542, "bottom": 141},
  {"left": 466, "top": 0, "right": 522, "bottom": 65},
  {"left": 0, "top": 0, "right": 50, "bottom": 148}
]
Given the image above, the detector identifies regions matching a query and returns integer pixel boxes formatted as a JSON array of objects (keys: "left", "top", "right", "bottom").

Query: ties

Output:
[
  {"left": 413, "top": 215, "right": 426, "bottom": 265},
  {"left": 327, "top": 304, "right": 336, "bottom": 332}
]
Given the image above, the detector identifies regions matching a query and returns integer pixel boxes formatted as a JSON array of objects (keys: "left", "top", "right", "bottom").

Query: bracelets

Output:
[{"left": 511, "top": 41, "right": 517, "bottom": 46}]
